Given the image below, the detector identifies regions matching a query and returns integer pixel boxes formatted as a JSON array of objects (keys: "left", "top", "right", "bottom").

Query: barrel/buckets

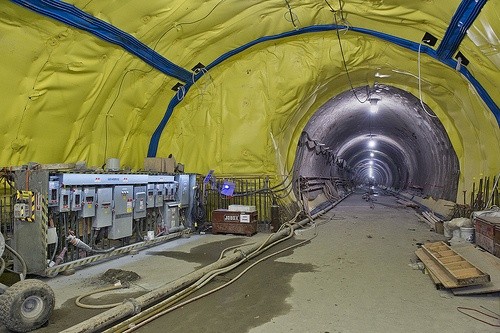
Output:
[
  {"left": 444, "top": 221, "right": 452, "bottom": 238},
  {"left": 453, "top": 228, "right": 460, "bottom": 241},
  {"left": 461, "top": 226, "right": 475, "bottom": 243}
]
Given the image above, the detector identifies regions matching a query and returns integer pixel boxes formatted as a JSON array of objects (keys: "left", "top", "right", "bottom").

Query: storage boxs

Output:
[
  {"left": 474, "top": 217, "right": 500, "bottom": 258},
  {"left": 212, "top": 208, "right": 258, "bottom": 238}
]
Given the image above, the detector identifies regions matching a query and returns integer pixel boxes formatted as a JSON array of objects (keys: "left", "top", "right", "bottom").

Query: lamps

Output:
[
  {"left": 366, "top": 98, "right": 382, "bottom": 114},
  {"left": 367, "top": 134, "right": 377, "bottom": 179}
]
